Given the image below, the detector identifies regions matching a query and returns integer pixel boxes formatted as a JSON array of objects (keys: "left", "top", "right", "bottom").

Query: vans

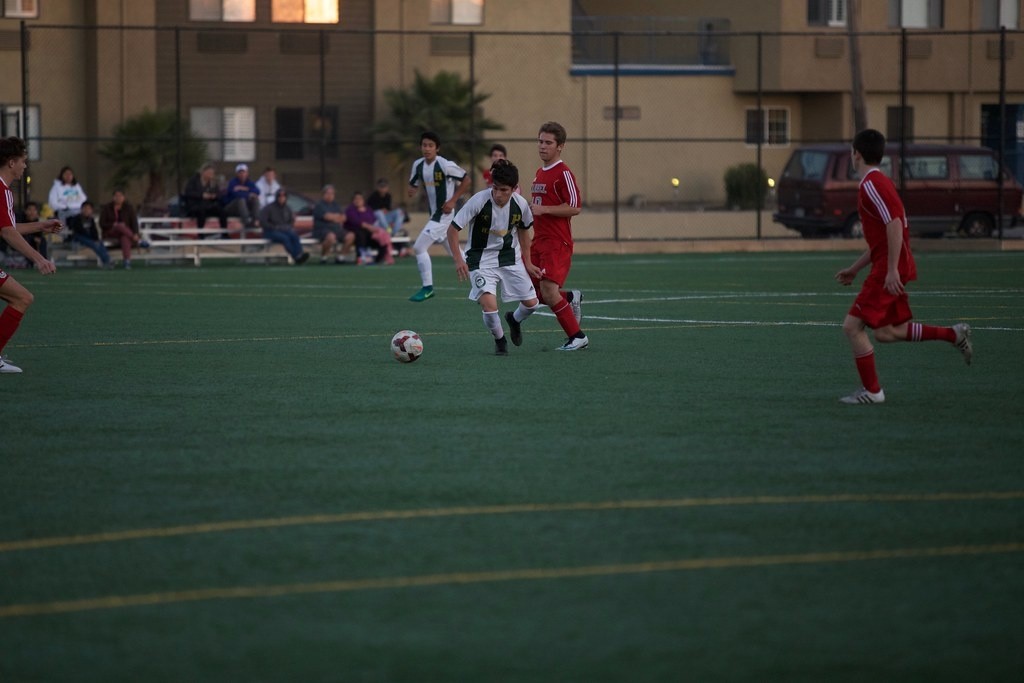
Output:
[{"left": 772, "top": 142, "right": 1024, "bottom": 239}]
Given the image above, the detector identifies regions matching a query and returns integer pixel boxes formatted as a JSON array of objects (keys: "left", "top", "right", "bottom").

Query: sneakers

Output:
[
  {"left": 840, "top": 387, "right": 886, "bottom": 404},
  {"left": 504, "top": 312, "right": 523, "bottom": 346},
  {"left": 409, "top": 288, "right": 435, "bottom": 302},
  {"left": 555, "top": 336, "right": 589, "bottom": 351},
  {"left": 569, "top": 290, "right": 583, "bottom": 325},
  {"left": 0, "top": 355, "right": 23, "bottom": 373},
  {"left": 496, "top": 335, "right": 508, "bottom": 356},
  {"left": 953, "top": 323, "right": 973, "bottom": 366}
]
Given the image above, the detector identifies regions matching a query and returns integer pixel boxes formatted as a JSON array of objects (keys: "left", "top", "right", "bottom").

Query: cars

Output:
[{"left": 160, "top": 181, "right": 316, "bottom": 245}]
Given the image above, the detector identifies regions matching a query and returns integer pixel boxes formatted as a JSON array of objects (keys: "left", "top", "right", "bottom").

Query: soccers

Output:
[{"left": 390, "top": 329, "right": 423, "bottom": 363}]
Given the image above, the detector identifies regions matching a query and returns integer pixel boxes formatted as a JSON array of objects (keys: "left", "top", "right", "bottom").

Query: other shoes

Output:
[
  {"left": 139, "top": 240, "right": 150, "bottom": 249},
  {"left": 296, "top": 251, "right": 309, "bottom": 265},
  {"left": 125, "top": 260, "right": 131, "bottom": 269},
  {"left": 319, "top": 256, "right": 328, "bottom": 265},
  {"left": 337, "top": 256, "right": 346, "bottom": 265},
  {"left": 108, "top": 258, "right": 116, "bottom": 271}
]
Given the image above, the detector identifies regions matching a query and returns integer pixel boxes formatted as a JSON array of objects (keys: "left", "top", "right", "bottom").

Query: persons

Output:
[
  {"left": 181, "top": 163, "right": 230, "bottom": 240},
  {"left": 312, "top": 184, "right": 355, "bottom": 264},
  {"left": 50, "top": 167, "right": 88, "bottom": 223},
  {"left": 346, "top": 177, "right": 404, "bottom": 266},
  {"left": 0, "top": 136, "right": 64, "bottom": 372},
  {"left": 405, "top": 132, "right": 472, "bottom": 301},
  {"left": 223, "top": 163, "right": 260, "bottom": 228},
  {"left": 254, "top": 166, "right": 281, "bottom": 214},
  {"left": 10, "top": 201, "right": 48, "bottom": 271},
  {"left": 446, "top": 159, "right": 540, "bottom": 357},
  {"left": 833, "top": 129, "right": 974, "bottom": 404},
  {"left": 99, "top": 190, "right": 151, "bottom": 271},
  {"left": 521, "top": 121, "right": 589, "bottom": 352},
  {"left": 482, "top": 144, "right": 521, "bottom": 195},
  {"left": 260, "top": 189, "right": 309, "bottom": 262},
  {"left": 68, "top": 202, "right": 118, "bottom": 269}
]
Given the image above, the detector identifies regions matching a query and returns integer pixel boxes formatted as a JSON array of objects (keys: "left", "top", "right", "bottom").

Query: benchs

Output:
[{"left": 67, "top": 216, "right": 409, "bottom": 267}]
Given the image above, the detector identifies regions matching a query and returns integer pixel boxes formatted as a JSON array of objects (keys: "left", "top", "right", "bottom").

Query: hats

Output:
[{"left": 236, "top": 163, "right": 248, "bottom": 173}]
